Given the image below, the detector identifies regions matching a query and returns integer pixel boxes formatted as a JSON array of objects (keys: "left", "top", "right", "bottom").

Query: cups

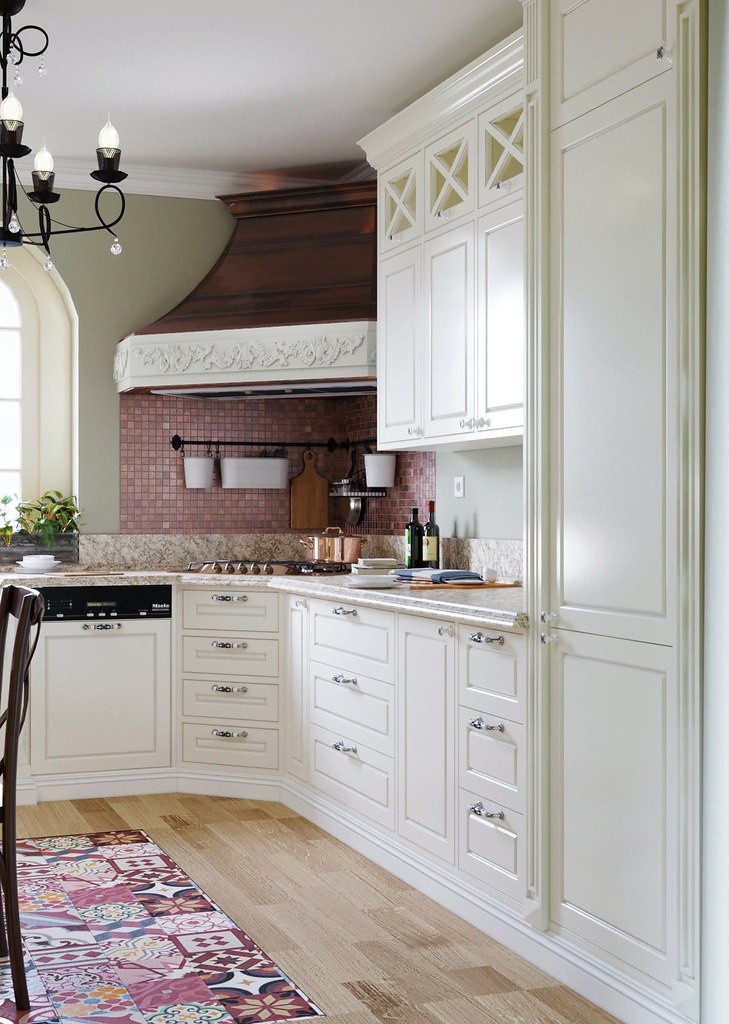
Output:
[
  {"left": 480, "top": 567, "right": 500, "bottom": 583},
  {"left": 181, "top": 456, "right": 214, "bottom": 488},
  {"left": 362, "top": 453, "right": 397, "bottom": 487}
]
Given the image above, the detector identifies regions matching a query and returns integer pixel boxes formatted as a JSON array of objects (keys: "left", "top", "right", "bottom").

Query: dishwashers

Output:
[{"left": 29, "top": 606, "right": 174, "bottom": 775}]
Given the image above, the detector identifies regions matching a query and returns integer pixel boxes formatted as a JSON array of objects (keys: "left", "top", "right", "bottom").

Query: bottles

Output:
[
  {"left": 405, "top": 508, "right": 424, "bottom": 569},
  {"left": 422, "top": 500, "right": 439, "bottom": 569}
]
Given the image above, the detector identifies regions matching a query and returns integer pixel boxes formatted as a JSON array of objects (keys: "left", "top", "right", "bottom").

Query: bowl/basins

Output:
[{"left": 22, "top": 555, "right": 55, "bottom": 561}]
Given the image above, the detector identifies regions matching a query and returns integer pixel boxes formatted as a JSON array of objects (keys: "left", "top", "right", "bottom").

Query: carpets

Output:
[{"left": 0, "top": 828, "right": 327, "bottom": 1024}]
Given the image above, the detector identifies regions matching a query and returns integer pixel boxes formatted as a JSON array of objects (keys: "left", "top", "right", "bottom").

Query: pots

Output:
[
  {"left": 339, "top": 447, "right": 361, "bottom": 526},
  {"left": 299, "top": 527, "right": 368, "bottom": 564}
]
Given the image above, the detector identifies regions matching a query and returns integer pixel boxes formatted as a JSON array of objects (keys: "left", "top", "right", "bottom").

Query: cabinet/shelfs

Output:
[
  {"left": 283, "top": 593, "right": 309, "bottom": 788},
  {"left": 309, "top": 597, "right": 397, "bottom": 840},
  {"left": 395, "top": 612, "right": 457, "bottom": 874},
  {"left": 377, "top": 242, "right": 422, "bottom": 450},
  {"left": 458, "top": 624, "right": 530, "bottom": 912},
  {"left": 175, "top": 585, "right": 283, "bottom": 777},
  {"left": 421, "top": 196, "right": 523, "bottom": 452}
]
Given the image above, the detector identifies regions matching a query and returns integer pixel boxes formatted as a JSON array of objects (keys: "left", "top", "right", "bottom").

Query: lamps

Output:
[{"left": 0, "top": 0, "right": 128, "bottom": 271}]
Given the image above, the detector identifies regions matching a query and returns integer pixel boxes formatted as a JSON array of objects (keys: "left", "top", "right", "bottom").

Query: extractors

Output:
[{"left": 149, "top": 381, "right": 377, "bottom": 401}]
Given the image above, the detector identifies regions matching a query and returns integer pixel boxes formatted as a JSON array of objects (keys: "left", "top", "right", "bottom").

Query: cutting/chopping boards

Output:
[
  {"left": 43, "top": 572, "right": 124, "bottom": 576},
  {"left": 291, "top": 450, "right": 328, "bottom": 529}
]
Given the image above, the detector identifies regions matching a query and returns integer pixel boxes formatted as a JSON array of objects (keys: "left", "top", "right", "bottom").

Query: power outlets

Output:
[{"left": 454, "top": 477, "right": 464, "bottom": 497}]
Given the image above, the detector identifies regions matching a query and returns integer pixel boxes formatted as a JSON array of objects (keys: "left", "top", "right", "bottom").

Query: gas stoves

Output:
[{"left": 166, "top": 559, "right": 352, "bottom": 575}]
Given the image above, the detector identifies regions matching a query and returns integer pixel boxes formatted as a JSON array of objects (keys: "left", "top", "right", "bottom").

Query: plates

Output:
[
  {"left": 445, "top": 580, "right": 486, "bottom": 584},
  {"left": 343, "top": 582, "right": 402, "bottom": 588},
  {"left": 16, "top": 561, "right": 62, "bottom": 568},
  {"left": 344, "top": 574, "right": 402, "bottom": 585}
]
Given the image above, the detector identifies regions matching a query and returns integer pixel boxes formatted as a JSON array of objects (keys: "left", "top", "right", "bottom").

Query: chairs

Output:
[{"left": 0, "top": 584, "right": 46, "bottom": 1011}]
